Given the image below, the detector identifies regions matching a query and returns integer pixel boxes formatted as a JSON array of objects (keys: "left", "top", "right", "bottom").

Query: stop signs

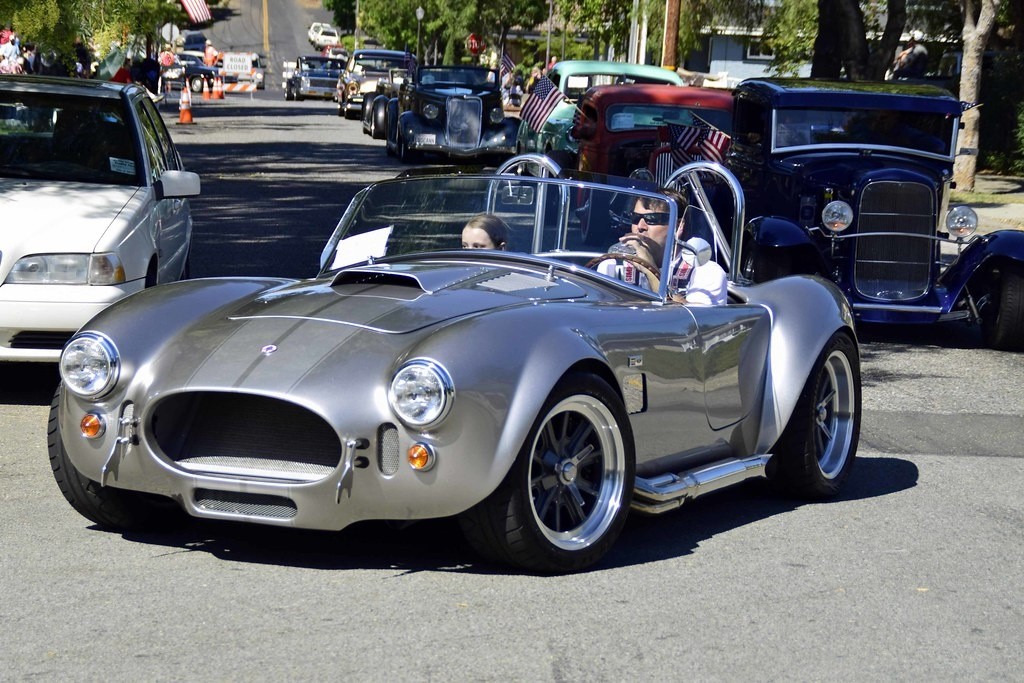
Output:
[{"left": 466, "top": 32, "right": 488, "bottom": 54}]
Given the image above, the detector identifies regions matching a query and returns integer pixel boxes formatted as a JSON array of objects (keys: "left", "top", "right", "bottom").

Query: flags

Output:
[
  {"left": 519, "top": 74, "right": 565, "bottom": 133},
  {"left": 496, "top": 49, "right": 516, "bottom": 77},
  {"left": 180, "top": 0, "right": 212, "bottom": 23},
  {"left": 404, "top": 43, "right": 416, "bottom": 77}
]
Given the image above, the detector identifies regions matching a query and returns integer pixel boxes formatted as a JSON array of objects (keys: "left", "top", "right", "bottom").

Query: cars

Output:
[
  {"left": 158, "top": 30, "right": 266, "bottom": 92},
  {"left": 687, "top": 74, "right": 1024, "bottom": 352},
  {"left": 307, "top": 22, "right": 348, "bottom": 61},
  {"left": 362, "top": 63, "right": 520, "bottom": 161},
  {"left": 1, "top": 72, "right": 202, "bottom": 387},
  {"left": 571, "top": 82, "right": 735, "bottom": 246},
  {"left": 516, "top": 56, "right": 686, "bottom": 220},
  {"left": 338, "top": 49, "right": 419, "bottom": 121},
  {"left": 283, "top": 55, "right": 347, "bottom": 104}
]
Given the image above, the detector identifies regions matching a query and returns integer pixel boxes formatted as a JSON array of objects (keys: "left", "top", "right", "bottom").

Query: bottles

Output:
[{"left": 608, "top": 237, "right": 643, "bottom": 256}]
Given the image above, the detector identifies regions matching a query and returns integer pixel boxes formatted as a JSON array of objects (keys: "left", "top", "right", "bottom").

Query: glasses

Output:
[{"left": 627, "top": 209, "right": 669, "bottom": 225}]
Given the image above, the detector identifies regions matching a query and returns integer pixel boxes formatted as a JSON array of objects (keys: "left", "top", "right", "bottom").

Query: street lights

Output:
[{"left": 416, "top": 6, "right": 425, "bottom": 64}]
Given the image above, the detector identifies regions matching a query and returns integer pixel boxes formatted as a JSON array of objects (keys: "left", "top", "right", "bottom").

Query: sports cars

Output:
[{"left": 45, "top": 150, "right": 865, "bottom": 577}]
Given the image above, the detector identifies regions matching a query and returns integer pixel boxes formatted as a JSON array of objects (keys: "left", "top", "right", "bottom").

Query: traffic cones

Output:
[
  {"left": 185, "top": 77, "right": 192, "bottom": 106},
  {"left": 217, "top": 77, "right": 224, "bottom": 99},
  {"left": 212, "top": 77, "right": 220, "bottom": 99},
  {"left": 202, "top": 78, "right": 211, "bottom": 99},
  {"left": 176, "top": 87, "right": 197, "bottom": 125}
]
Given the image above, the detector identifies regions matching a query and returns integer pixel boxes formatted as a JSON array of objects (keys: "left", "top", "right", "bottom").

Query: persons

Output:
[
  {"left": 895, "top": 29, "right": 928, "bottom": 77},
  {"left": 202, "top": 39, "right": 219, "bottom": 93},
  {"left": 157, "top": 43, "right": 174, "bottom": 95},
  {"left": 596, "top": 186, "right": 728, "bottom": 305},
  {"left": 487, "top": 55, "right": 557, "bottom": 106},
  {"left": 462, "top": 213, "right": 507, "bottom": 251}
]
